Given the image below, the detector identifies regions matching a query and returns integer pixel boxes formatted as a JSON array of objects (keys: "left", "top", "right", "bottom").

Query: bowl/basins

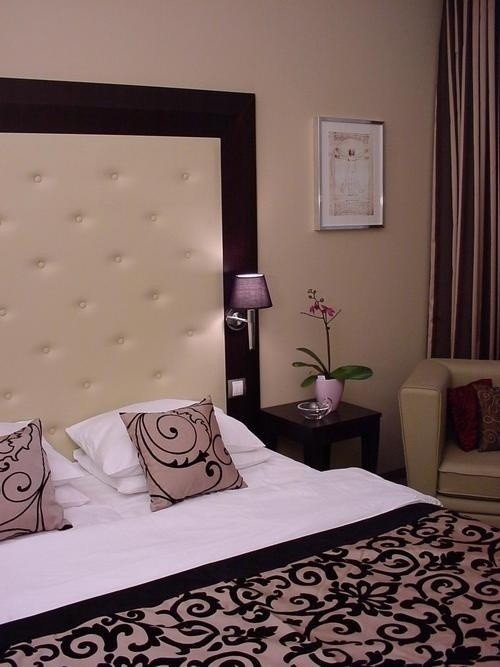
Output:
[{"left": 298, "top": 401, "right": 330, "bottom": 419}]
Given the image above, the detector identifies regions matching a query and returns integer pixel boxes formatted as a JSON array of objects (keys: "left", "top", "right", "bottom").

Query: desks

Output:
[{"left": 260, "top": 397, "right": 383, "bottom": 472}]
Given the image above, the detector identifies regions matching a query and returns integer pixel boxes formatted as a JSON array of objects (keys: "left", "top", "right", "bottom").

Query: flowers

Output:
[{"left": 291, "top": 286, "right": 376, "bottom": 388}]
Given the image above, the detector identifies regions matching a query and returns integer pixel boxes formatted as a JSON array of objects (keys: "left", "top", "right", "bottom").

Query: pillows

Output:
[
  {"left": 120, "top": 393, "right": 251, "bottom": 512},
  {"left": 473, "top": 382, "right": 500, "bottom": 453},
  {"left": 1, "top": 421, "right": 86, "bottom": 487},
  {"left": 72, "top": 447, "right": 273, "bottom": 495},
  {"left": 1, "top": 417, "right": 73, "bottom": 541},
  {"left": 448, "top": 378, "right": 496, "bottom": 452},
  {"left": 53, "top": 483, "right": 91, "bottom": 510},
  {"left": 65, "top": 398, "right": 268, "bottom": 478}
]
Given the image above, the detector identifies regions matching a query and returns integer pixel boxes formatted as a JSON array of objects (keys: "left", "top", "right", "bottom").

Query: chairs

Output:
[{"left": 395, "top": 358, "right": 500, "bottom": 526}]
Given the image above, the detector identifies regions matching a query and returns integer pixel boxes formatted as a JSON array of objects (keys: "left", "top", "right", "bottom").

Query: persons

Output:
[{"left": 333, "top": 144, "right": 372, "bottom": 200}]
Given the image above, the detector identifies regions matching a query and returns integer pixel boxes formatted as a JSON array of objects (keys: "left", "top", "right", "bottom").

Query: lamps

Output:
[{"left": 224, "top": 271, "right": 274, "bottom": 353}]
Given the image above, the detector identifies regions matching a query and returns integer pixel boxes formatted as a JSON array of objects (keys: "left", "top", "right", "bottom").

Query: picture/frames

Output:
[
  {"left": 313, "top": 375, "right": 344, "bottom": 414},
  {"left": 313, "top": 113, "right": 387, "bottom": 232}
]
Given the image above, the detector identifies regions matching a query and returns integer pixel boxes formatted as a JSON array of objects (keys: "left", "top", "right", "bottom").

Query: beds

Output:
[{"left": 1, "top": 72, "right": 500, "bottom": 666}]
